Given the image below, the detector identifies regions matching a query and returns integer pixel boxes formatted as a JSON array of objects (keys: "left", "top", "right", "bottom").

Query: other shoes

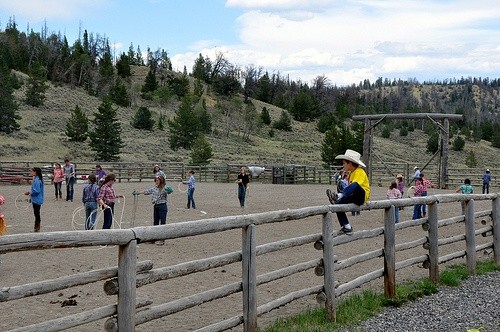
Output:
[{"left": 156, "top": 241, "right": 164, "bottom": 245}]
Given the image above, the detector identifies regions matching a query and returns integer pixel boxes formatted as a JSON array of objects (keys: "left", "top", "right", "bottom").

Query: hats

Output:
[
  {"left": 153, "top": 164, "right": 161, "bottom": 169},
  {"left": 413, "top": 166, "right": 419, "bottom": 170},
  {"left": 486, "top": 169, "right": 490, "bottom": 171},
  {"left": 335, "top": 149, "right": 366, "bottom": 168},
  {"left": 396, "top": 173, "right": 404, "bottom": 179}
]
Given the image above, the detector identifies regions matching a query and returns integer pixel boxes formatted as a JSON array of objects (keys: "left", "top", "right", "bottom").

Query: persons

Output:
[
  {"left": 336, "top": 171, "right": 344, "bottom": 184},
  {"left": 385, "top": 181, "right": 402, "bottom": 224},
  {"left": 153, "top": 164, "right": 166, "bottom": 179},
  {"left": 63, "top": 159, "right": 75, "bottom": 202},
  {"left": 94, "top": 165, "right": 107, "bottom": 186},
  {"left": 51, "top": 163, "right": 64, "bottom": 200},
  {"left": 419, "top": 174, "right": 437, "bottom": 216},
  {"left": 411, "top": 166, "right": 420, "bottom": 185},
  {"left": 183, "top": 170, "right": 196, "bottom": 209},
  {"left": 0, "top": 194, "right": 8, "bottom": 237},
  {"left": 337, "top": 174, "right": 349, "bottom": 194},
  {"left": 235, "top": 167, "right": 250, "bottom": 209},
  {"left": 411, "top": 178, "right": 424, "bottom": 220},
  {"left": 96, "top": 172, "right": 123, "bottom": 247},
  {"left": 82, "top": 175, "right": 100, "bottom": 230},
  {"left": 132, "top": 175, "right": 174, "bottom": 245},
  {"left": 455, "top": 179, "right": 474, "bottom": 215},
  {"left": 481, "top": 169, "right": 493, "bottom": 195},
  {"left": 24, "top": 166, "right": 44, "bottom": 232},
  {"left": 326, "top": 149, "right": 370, "bottom": 235},
  {"left": 395, "top": 174, "right": 404, "bottom": 210},
  {"left": 351, "top": 211, "right": 360, "bottom": 216}
]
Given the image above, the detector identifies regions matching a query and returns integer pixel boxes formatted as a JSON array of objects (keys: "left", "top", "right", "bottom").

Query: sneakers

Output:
[
  {"left": 337, "top": 227, "right": 353, "bottom": 235},
  {"left": 326, "top": 189, "right": 338, "bottom": 205}
]
